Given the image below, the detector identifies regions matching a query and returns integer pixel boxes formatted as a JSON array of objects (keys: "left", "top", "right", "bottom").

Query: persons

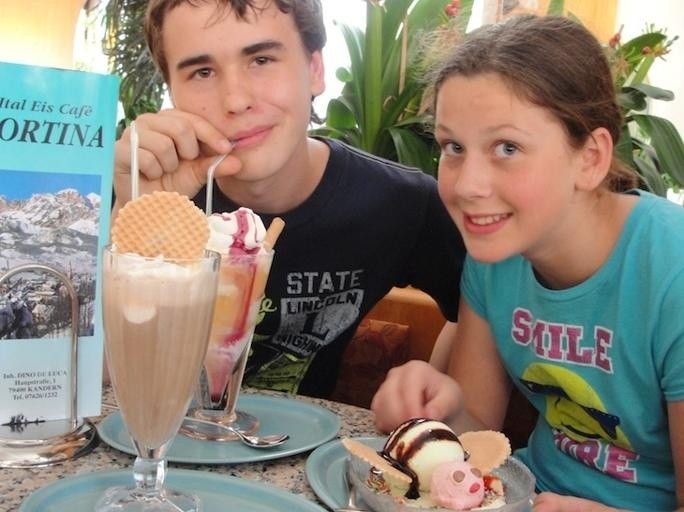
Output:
[
  {"left": 103, "top": 0, "right": 467, "bottom": 400},
  {"left": 370, "top": 14, "right": 684, "bottom": 512}
]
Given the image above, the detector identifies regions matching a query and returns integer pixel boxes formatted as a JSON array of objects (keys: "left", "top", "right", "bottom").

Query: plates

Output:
[
  {"left": 306, "top": 433, "right": 380, "bottom": 510},
  {"left": 98, "top": 391, "right": 343, "bottom": 467},
  {"left": 17, "top": 467, "right": 325, "bottom": 510}
]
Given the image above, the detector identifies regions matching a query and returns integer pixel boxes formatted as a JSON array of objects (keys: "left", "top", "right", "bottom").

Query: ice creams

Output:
[
  {"left": 340, "top": 415, "right": 511, "bottom": 512},
  {"left": 206, "top": 207, "right": 269, "bottom": 402}
]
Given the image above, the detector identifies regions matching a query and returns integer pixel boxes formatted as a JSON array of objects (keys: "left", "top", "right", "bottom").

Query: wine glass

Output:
[{"left": 100, "top": 246, "right": 200, "bottom": 512}]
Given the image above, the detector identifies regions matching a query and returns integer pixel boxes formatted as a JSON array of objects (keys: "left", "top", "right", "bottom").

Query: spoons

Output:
[{"left": 99, "top": 400, "right": 290, "bottom": 450}]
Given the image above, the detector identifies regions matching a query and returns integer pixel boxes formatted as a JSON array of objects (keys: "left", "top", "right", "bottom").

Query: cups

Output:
[{"left": 178, "top": 253, "right": 261, "bottom": 445}]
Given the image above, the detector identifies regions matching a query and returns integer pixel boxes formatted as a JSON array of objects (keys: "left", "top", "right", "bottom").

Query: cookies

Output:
[{"left": 112, "top": 191, "right": 211, "bottom": 260}]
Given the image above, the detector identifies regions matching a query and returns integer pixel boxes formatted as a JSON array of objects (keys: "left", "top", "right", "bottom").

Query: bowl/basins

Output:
[{"left": 348, "top": 448, "right": 535, "bottom": 510}]
